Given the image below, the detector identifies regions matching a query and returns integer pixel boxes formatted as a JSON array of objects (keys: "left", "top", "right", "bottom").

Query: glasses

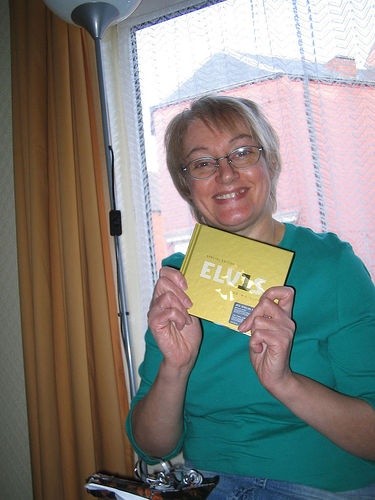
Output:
[{"left": 182, "top": 145, "right": 264, "bottom": 180}]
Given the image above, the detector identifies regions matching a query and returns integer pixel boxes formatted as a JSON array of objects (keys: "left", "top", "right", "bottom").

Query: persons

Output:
[{"left": 124, "top": 94, "right": 375, "bottom": 500}]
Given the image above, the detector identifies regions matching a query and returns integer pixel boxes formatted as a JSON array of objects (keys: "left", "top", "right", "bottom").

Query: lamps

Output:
[{"left": 45, "top": 0, "right": 149, "bottom": 482}]
